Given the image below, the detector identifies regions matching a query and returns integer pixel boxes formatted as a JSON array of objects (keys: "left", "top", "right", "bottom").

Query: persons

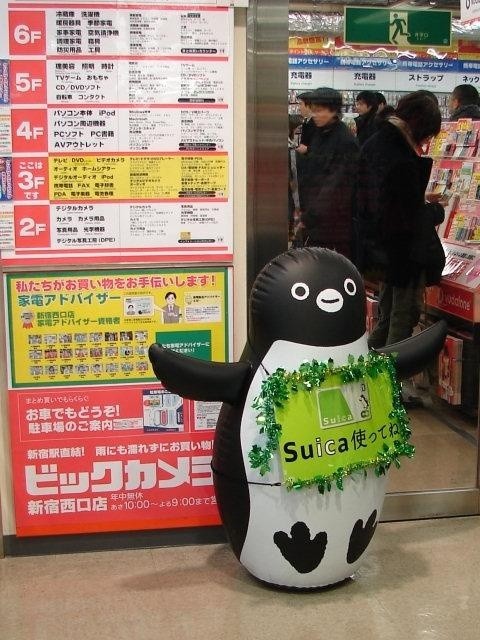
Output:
[
  {"left": 290, "top": 87, "right": 448, "bottom": 408},
  {"left": 448, "top": 85, "right": 479, "bottom": 120}
]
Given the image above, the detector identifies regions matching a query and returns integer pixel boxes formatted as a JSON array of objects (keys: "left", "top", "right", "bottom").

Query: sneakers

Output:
[{"left": 398, "top": 384, "right": 434, "bottom": 409}]
